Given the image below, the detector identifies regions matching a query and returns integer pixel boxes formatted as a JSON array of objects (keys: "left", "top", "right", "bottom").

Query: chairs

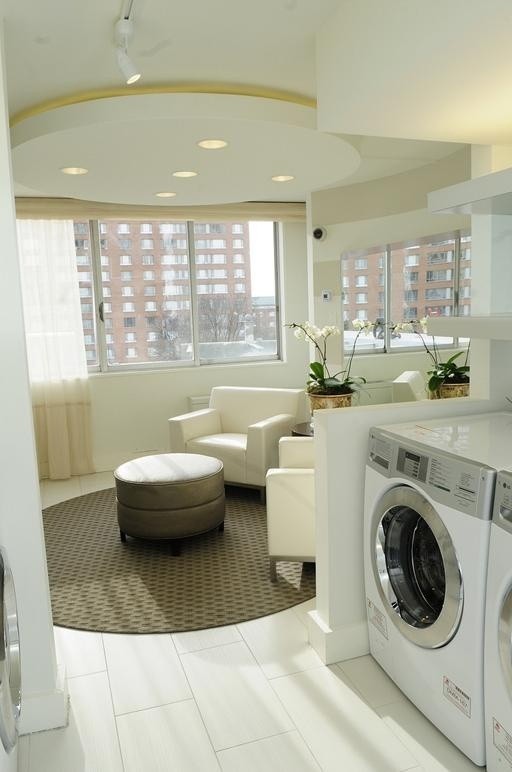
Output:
[
  {"left": 168, "top": 386, "right": 303, "bottom": 507},
  {"left": 265, "top": 436, "right": 316, "bottom": 583}
]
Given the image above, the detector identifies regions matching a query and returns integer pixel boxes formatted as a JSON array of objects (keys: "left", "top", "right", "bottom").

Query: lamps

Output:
[{"left": 113, "top": 43, "right": 142, "bottom": 87}]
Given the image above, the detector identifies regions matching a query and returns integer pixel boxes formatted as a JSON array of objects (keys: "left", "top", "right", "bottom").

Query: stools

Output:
[{"left": 114, "top": 452, "right": 228, "bottom": 556}]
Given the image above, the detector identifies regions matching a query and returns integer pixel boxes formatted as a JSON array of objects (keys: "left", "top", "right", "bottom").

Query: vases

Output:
[
  {"left": 439, "top": 382, "right": 470, "bottom": 401},
  {"left": 305, "top": 387, "right": 355, "bottom": 432}
]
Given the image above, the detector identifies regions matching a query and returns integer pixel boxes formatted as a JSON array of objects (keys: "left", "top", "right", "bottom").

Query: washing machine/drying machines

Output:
[
  {"left": 360, "top": 411, "right": 512, "bottom": 767},
  {"left": 484, "top": 470, "right": 512, "bottom": 766}
]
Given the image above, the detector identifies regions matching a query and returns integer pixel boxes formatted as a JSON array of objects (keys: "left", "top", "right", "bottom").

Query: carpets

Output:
[{"left": 41, "top": 484, "right": 317, "bottom": 635}]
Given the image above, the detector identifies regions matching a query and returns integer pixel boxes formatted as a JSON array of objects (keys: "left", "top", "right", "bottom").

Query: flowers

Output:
[
  {"left": 389, "top": 318, "right": 473, "bottom": 390},
  {"left": 283, "top": 319, "right": 389, "bottom": 399}
]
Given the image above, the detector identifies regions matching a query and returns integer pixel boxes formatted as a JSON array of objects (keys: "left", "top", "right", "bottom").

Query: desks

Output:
[{"left": 291, "top": 421, "right": 314, "bottom": 438}]
